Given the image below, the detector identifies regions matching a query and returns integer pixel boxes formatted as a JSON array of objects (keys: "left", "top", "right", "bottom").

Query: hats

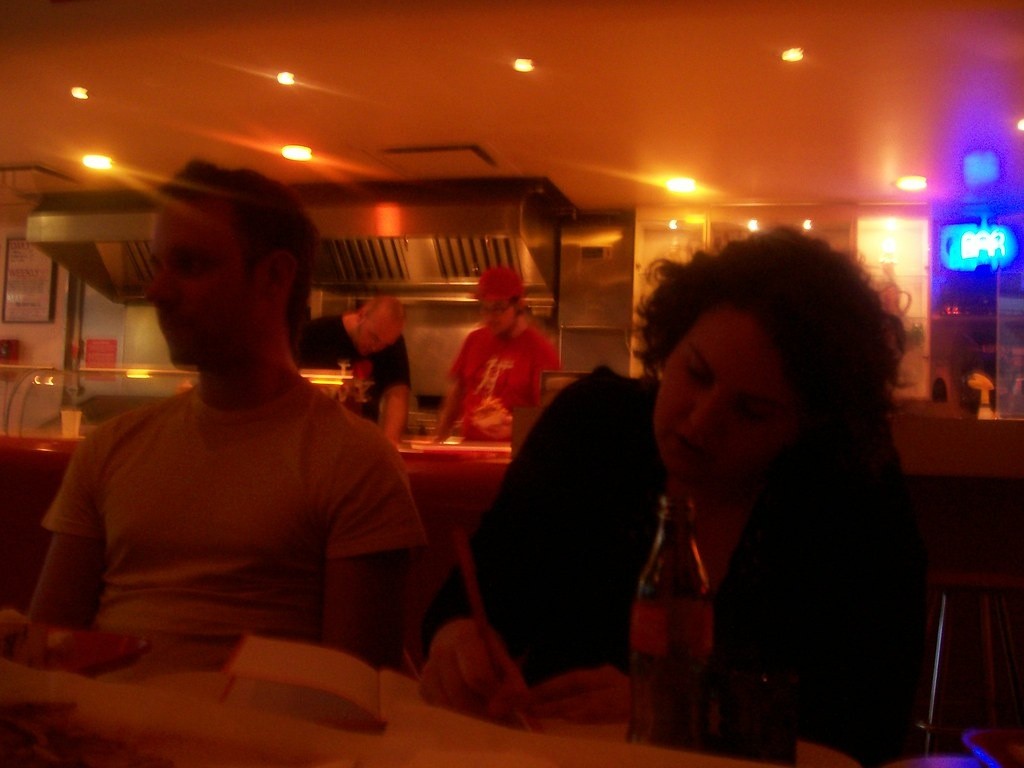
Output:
[{"left": 468, "top": 265, "right": 524, "bottom": 301}]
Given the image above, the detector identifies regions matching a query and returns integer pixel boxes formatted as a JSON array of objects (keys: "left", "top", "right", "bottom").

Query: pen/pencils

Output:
[{"left": 450, "top": 523, "right": 526, "bottom": 726}]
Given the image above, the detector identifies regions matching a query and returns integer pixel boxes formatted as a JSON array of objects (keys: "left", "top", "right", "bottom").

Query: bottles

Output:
[{"left": 627, "top": 496, "right": 718, "bottom": 752}]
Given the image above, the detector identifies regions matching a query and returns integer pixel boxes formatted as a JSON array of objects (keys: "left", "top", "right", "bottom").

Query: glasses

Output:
[{"left": 480, "top": 302, "right": 511, "bottom": 312}]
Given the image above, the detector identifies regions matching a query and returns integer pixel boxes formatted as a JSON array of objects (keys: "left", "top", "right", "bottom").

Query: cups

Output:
[{"left": 59, "top": 409, "right": 82, "bottom": 438}]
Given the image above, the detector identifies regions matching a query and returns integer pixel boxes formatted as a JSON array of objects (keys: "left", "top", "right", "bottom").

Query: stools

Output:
[{"left": 914, "top": 570, "right": 1024, "bottom": 759}]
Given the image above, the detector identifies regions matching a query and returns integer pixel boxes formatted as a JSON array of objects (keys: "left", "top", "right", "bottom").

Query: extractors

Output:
[{"left": 27, "top": 177, "right": 573, "bottom": 317}]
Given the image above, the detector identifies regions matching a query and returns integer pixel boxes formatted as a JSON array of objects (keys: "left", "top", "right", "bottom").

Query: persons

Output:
[
  {"left": 419, "top": 221, "right": 928, "bottom": 768},
  {"left": 27, "top": 157, "right": 432, "bottom": 667},
  {"left": 298, "top": 295, "right": 411, "bottom": 443},
  {"left": 436, "top": 265, "right": 562, "bottom": 446}
]
